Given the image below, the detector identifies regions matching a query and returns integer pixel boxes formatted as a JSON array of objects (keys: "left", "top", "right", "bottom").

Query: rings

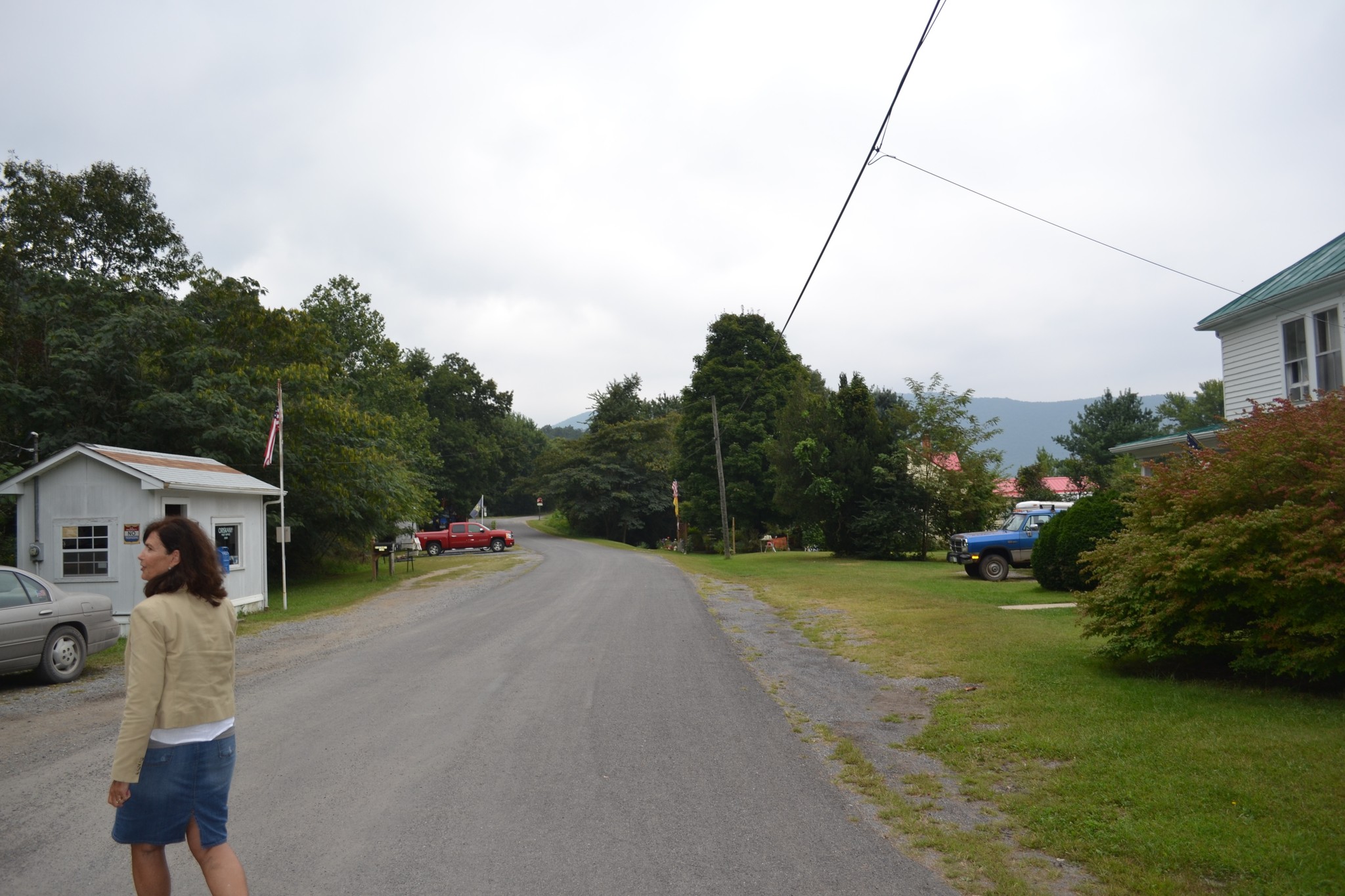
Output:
[{"left": 117, "top": 798, "right": 122, "bottom": 803}]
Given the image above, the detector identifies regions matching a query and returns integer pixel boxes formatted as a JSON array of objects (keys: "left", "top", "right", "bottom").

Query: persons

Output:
[
  {"left": 108, "top": 517, "right": 249, "bottom": 896},
  {"left": 432, "top": 518, "right": 439, "bottom": 530}
]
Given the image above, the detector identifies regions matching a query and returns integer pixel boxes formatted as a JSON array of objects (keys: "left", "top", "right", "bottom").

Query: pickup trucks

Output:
[{"left": 415, "top": 523, "right": 515, "bottom": 557}]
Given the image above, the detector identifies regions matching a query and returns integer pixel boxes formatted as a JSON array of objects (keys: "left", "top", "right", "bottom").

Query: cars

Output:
[{"left": 0, "top": 566, "right": 120, "bottom": 684}]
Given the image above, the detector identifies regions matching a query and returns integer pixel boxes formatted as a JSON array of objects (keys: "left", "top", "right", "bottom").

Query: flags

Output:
[
  {"left": 470, "top": 498, "right": 482, "bottom": 519},
  {"left": 672, "top": 478, "right": 679, "bottom": 516},
  {"left": 264, "top": 403, "right": 283, "bottom": 467}
]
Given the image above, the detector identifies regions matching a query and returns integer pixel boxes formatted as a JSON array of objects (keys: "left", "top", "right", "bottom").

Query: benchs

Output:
[{"left": 759, "top": 539, "right": 774, "bottom": 553}]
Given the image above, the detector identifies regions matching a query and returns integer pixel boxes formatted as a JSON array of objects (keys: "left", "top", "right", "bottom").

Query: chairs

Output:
[{"left": 765, "top": 536, "right": 777, "bottom": 553}]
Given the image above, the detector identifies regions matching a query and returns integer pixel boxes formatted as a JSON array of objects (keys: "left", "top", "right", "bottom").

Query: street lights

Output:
[{"left": 29, "top": 431, "right": 39, "bottom": 574}]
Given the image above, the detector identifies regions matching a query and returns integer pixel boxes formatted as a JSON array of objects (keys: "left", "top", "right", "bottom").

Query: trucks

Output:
[
  {"left": 946, "top": 504, "right": 1066, "bottom": 582},
  {"left": 1004, "top": 501, "right": 1075, "bottom": 527}
]
